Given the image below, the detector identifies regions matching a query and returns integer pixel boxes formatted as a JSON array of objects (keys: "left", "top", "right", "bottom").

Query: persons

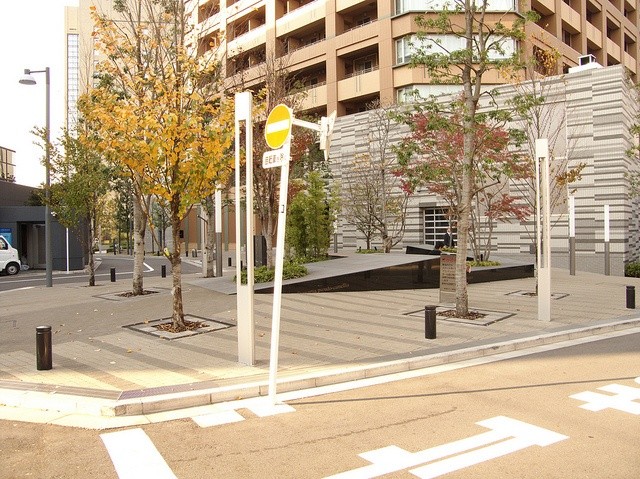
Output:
[{"left": 443, "top": 228, "right": 455, "bottom": 248}]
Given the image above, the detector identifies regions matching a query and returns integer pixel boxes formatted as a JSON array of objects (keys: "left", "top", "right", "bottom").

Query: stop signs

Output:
[{"left": 263, "top": 104, "right": 291, "bottom": 149}]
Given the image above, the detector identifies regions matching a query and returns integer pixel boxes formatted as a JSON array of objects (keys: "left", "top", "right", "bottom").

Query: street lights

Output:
[{"left": 19, "top": 66, "right": 53, "bottom": 288}]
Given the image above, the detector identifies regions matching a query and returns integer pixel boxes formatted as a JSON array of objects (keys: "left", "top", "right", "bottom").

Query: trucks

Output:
[{"left": 0, "top": 235, "right": 21, "bottom": 274}]
